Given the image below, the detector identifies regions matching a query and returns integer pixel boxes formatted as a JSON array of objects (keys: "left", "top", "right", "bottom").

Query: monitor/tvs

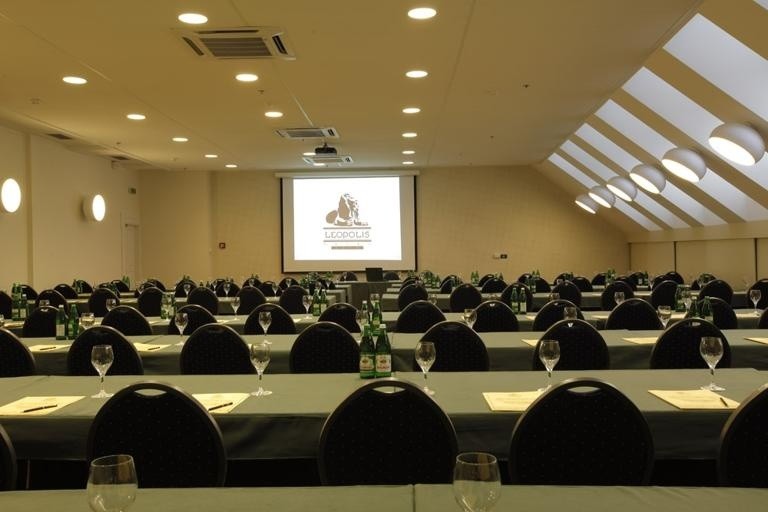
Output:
[{"left": 366, "top": 268, "right": 383, "bottom": 282}]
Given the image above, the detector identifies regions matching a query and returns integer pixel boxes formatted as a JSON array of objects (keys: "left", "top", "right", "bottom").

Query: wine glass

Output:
[
  {"left": 749, "top": 290, "right": 762, "bottom": 316},
  {"left": 699, "top": 336, "right": 730, "bottom": 394},
  {"left": 90, "top": 345, "right": 114, "bottom": 400},
  {"left": 539, "top": 342, "right": 562, "bottom": 393},
  {"left": 416, "top": 339, "right": 437, "bottom": 397},
  {"left": 250, "top": 344, "right": 272, "bottom": 396}
]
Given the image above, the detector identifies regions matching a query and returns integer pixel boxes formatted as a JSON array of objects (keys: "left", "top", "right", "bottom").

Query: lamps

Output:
[
  {"left": 604, "top": 175, "right": 637, "bottom": 203},
  {"left": 628, "top": 164, "right": 665, "bottom": 195},
  {"left": 586, "top": 185, "right": 616, "bottom": 210},
  {"left": 0, "top": 176, "right": 21, "bottom": 215},
  {"left": 82, "top": 192, "right": 106, "bottom": 223},
  {"left": 660, "top": 147, "right": 707, "bottom": 183},
  {"left": 708, "top": 122, "right": 765, "bottom": 167},
  {"left": 571, "top": 193, "right": 600, "bottom": 215}
]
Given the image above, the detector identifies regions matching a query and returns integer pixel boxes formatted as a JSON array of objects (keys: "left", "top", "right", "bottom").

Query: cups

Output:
[
  {"left": 454, "top": 452, "right": 501, "bottom": 510},
  {"left": 84, "top": 455, "right": 140, "bottom": 511}
]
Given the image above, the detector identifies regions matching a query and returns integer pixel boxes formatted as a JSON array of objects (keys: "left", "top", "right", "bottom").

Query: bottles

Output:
[
  {"left": 377, "top": 323, "right": 391, "bottom": 377},
  {"left": 9, "top": 268, "right": 717, "bottom": 342},
  {"left": 359, "top": 322, "right": 375, "bottom": 376}
]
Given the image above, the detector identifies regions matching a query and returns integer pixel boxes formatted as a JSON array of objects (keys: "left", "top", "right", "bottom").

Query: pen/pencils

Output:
[
  {"left": 209, "top": 402, "right": 233, "bottom": 410},
  {"left": 148, "top": 347, "right": 160, "bottom": 350},
  {"left": 40, "top": 346, "right": 56, "bottom": 350},
  {"left": 24, "top": 404, "right": 58, "bottom": 413},
  {"left": 720, "top": 397, "right": 728, "bottom": 406}
]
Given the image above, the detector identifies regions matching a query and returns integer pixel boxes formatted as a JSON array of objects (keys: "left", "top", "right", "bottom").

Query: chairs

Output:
[{"left": 0, "top": 271, "right": 767, "bottom": 512}]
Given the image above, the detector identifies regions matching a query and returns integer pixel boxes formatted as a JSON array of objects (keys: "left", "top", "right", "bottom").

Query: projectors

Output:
[{"left": 314, "top": 147, "right": 337, "bottom": 157}]
